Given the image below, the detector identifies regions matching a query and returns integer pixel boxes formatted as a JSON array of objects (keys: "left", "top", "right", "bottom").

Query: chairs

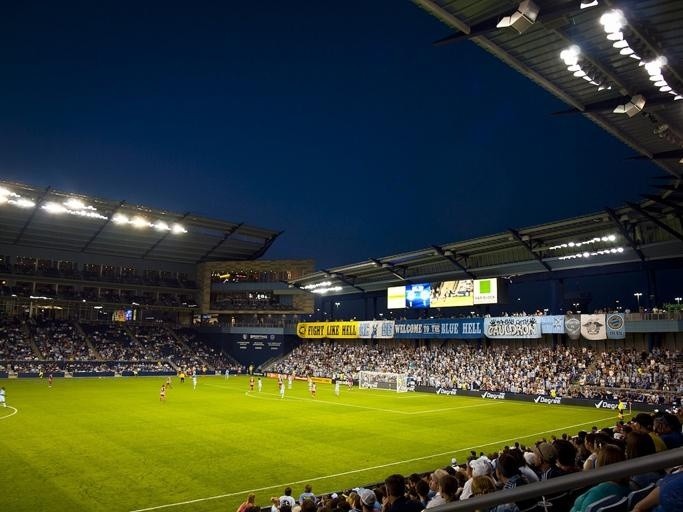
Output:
[{"left": 522, "top": 461, "right": 682, "bottom": 512}]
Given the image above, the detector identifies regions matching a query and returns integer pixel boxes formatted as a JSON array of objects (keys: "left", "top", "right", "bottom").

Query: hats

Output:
[
  {"left": 528, "top": 443, "right": 557, "bottom": 465},
  {"left": 632, "top": 413, "right": 654, "bottom": 427},
  {"left": 523, "top": 452, "right": 542, "bottom": 466},
  {"left": 358, "top": 488, "right": 377, "bottom": 506},
  {"left": 470, "top": 459, "right": 492, "bottom": 475},
  {"left": 430, "top": 469, "right": 448, "bottom": 480}
]
